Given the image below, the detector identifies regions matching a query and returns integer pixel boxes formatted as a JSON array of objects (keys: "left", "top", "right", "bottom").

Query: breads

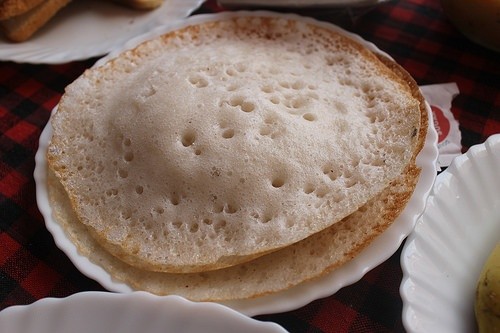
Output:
[
  {"left": 0, "top": 0, "right": 163, "bottom": 44},
  {"left": 473, "top": 240, "right": 500, "bottom": 333}
]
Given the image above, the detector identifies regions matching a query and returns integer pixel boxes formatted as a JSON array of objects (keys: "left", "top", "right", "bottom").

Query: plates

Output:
[
  {"left": 400, "top": 134, "right": 500, "bottom": 333},
  {"left": 33, "top": 10, "right": 439, "bottom": 318},
  {"left": 0, "top": 291, "right": 290, "bottom": 333},
  {"left": 0, "top": 0, "right": 209, "bottom": 66}
]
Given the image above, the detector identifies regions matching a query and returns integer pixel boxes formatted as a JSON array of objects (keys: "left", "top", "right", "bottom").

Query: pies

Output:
[{"left": 44, "top": 16, "right": 431, "bottom": 303}]
